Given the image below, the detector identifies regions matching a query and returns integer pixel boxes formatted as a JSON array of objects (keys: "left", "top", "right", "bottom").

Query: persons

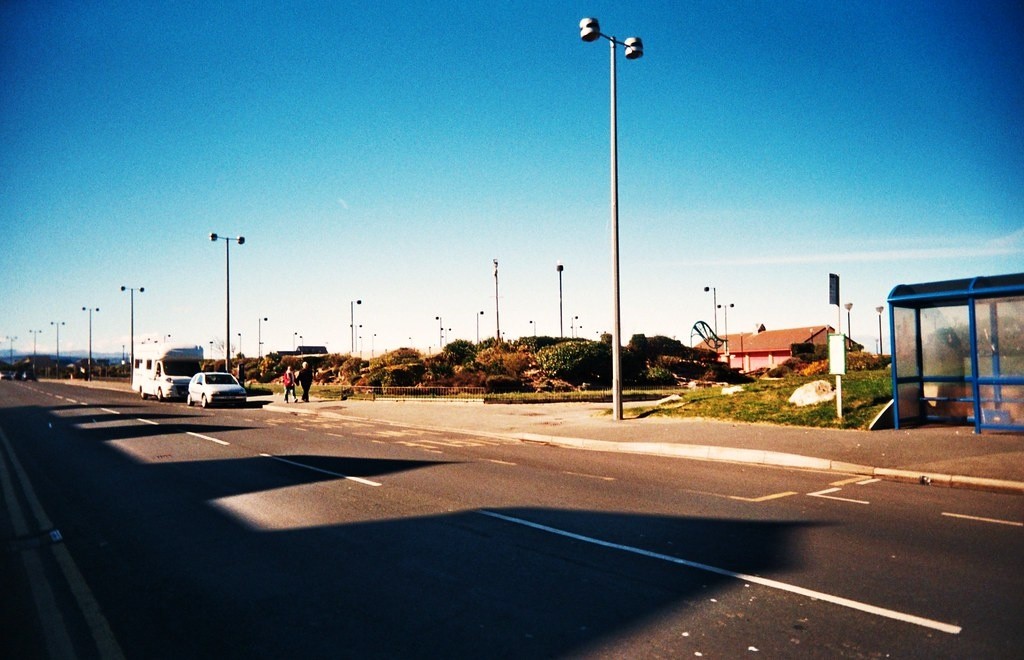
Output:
[
  {"left": 283, "top": 366, "right": 298, "bottom": 403},
  {"left": 296, "top": 362, "right": 313, "bottom": 402}
]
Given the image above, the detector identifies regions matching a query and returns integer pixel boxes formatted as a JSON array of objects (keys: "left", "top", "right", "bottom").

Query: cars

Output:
[
  {"left": 0, "top": 364, "right": 34, "bottom": 381},
  {"left": 186, "top": 371, "right": 247, "bottom": 408}
]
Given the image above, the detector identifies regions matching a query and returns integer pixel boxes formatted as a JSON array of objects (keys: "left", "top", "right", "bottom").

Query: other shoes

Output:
[
  {"left": 284, "top": 399, "right": 288, "bottom": 402},
  {"left": 302, "top": 398, "right": 308, "bottom": 402},
  {"left": 294, "top": 399, "right": 298, "bottom": 402}
]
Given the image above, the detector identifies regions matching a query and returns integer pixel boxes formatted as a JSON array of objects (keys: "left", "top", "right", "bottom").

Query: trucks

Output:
[{"left": 131, "top": 356, "right": 192, "bottom": 402}]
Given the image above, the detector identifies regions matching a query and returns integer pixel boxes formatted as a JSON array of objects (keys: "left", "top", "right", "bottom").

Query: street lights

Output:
[
  {"left": 238, "top": 333, "right": 242, "bottom": 358},
  {"left": 293, "top": 332, "right": 297, "bottom": 356},
  {"left": 351, "top": 300, "right": 362, "bottom": 352},
  {"left": 209, "top": 342, "right": 213, "bottom": 360},
  {"left": 29, "top": 330, "right": 42, "bottom": 375},
  {"left": 571, "top": 316, "right": 578, "bottom": 338},
  {"left": 477, "top": 311, "right": 484, "bottom": 342},
  {"left": 356, "top": 325, "right": 362, "bottom": 354},
  {"left": 716, "top": 304, "right": 735, "bottom": 352},
  {"left": 82, "top": 307, "right": 99, "bottom": 381},
  {"left": 876, "top": 306, "right": 884, "bottom": 354},
  {"left": 6, "top": 336, "right": 17, "bottom": 366},
  {"left": 557, "top": 265, "right": 563, "bottom": 338},
  {"left": 300, "top": 336, "right": 302, "bottom": 355},
  {"left": 845, "top": 303, "right": 853, "bottom": 350},
  {"left": 208, "top": 233, "right": 245, "bottom": 373},
  {"left": 436, "top": 317, "right": 442, "bottom": 350},
  {"left": 704, "top": 286, "right": 718, "bottom": 356},
  {"left": 164, "top": 334, "right": 171, "bottom": 354},
  {"left": 494, "top": 259, "right": 500, "bottom": 338},
  {"left": 50, "top": 322, "right": 65, "bottom": 378},
  {"left": 579, "top": 17, "right": 644, "bottom": 421},
  {"left": 121, "top": 286, "right": 144, "bottom": 383},
  {"left": 259, "top": 317, "right": 268, "bottom": 360},
  {"left": 530, "top": 321, "right": 536, "bottom": 336}
]
